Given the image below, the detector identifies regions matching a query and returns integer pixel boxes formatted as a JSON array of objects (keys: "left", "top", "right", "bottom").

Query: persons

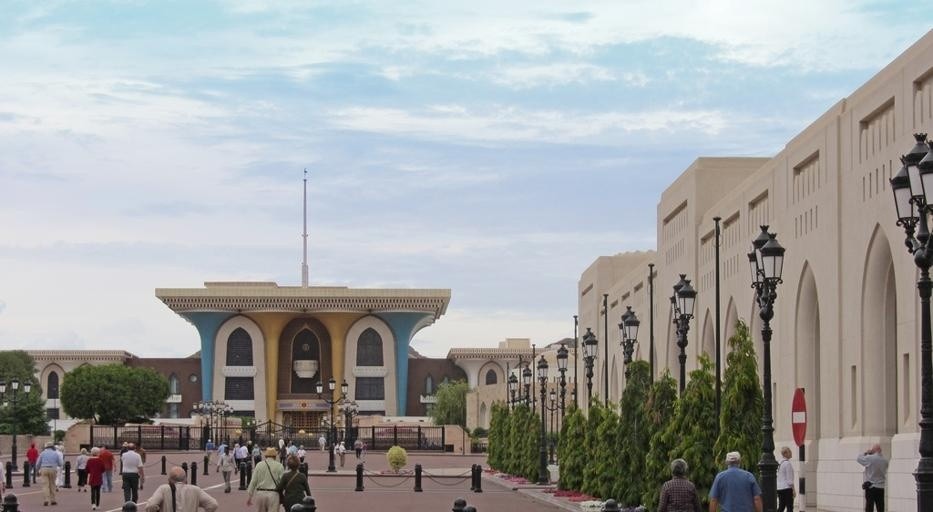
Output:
[
  {"left": 709, "top": 452, "right": 762, "bottom": 512},
  {"left": 363, "top": 441, "right": 369, "bottom": 456},
  {"left": 658, "top": 460, "right": 700, "bottom": 512},
  {"left": 0, "top": 452, "right": 6, "bottom": 505},
  {"left": 775, "top": 446, "right": 796, "bottom": 512},
  {"left": 206, "top": 438, "right": 311, "bottom": 512},
  {"left": 144, "top": 466, "right": 219, "bottom": 512},
  {"left": 334, "top": 441, "right": 340, "bottom": 465},
  {"left": 338, "top": 441, "right": 349, "bottom": 467},
  {"left": 318, "top": 434, "right": 326, "bottom": 452},
  {"left": 28, "top": 441, "right": 145, "bottom": 507},
  {"left": 858, "top": 444, "right": 888, "bottom": 512},
  {"left": 354, "top": 437, "right": 363, "bottom": 458}
]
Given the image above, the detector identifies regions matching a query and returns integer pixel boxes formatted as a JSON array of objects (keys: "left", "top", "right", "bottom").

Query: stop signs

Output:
[{"left": 791, "top": 387, "right": 807, "bottom": 446}]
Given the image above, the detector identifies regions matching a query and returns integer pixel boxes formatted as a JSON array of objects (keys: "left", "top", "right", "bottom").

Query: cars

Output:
[{"left": 471, "top": 433, "right": 488, "bottom": 453}]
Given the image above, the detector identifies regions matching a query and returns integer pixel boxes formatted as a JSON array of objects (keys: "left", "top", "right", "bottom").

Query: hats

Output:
[
  {"left": 727, "top": 452, "right": 740, "bottom": 462},
  {"left": 265, "top": 447, "right": 277, "bottom": 456}
]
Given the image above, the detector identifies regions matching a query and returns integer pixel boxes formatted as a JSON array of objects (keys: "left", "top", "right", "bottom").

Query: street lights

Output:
[
  {"left": 521, "top": 364, "right": 532, "bottom": 407},
  {"left": 745, "top": 225, "right": 787, "bottom": 511},
  {"left": 581, "top": 327, "right": 599, "bottom": 416},
  {"left": 555, "top": 342, "right": 570, "bottom": 430},
  {"left": 570, "top": 387, "right": 575, "bottom": 401},
  {"left": 888, "top": 131, "right": 932, "bottom": 510},
  {"left": 0, "top": 376, "right": 33, "bottom": 473},
  {"left": 314, "top": 376, "right": 348, "bottom": 472},
  {"left": 536, "top": 354, "right": 549, "bottom": 483},
  {"left": 319, "top": 397, "right": 360, "bottom": 450},
  {"left": 245, "top": 418, "right": 261, "bottom": 447},
  {"left": 616, "top": 305, "right": 642, "bottom": 387},
  {"left": 550, "top": 388, "right": 556, "bottom": 465},
  {"left": 668, "top": 272, "right": 699, "bottom": 398},
  {"left": 507, "top": 371, "right": 518, "bottom": 412},
  {"left": 192, "top": 398, "right": 233, "bottom": 450},
  {"left": 319, "top": 414, "right": 340, "bottom": 428},
  {"left": 51, "top": 385, "right": 57, "bottom": 445}
]
